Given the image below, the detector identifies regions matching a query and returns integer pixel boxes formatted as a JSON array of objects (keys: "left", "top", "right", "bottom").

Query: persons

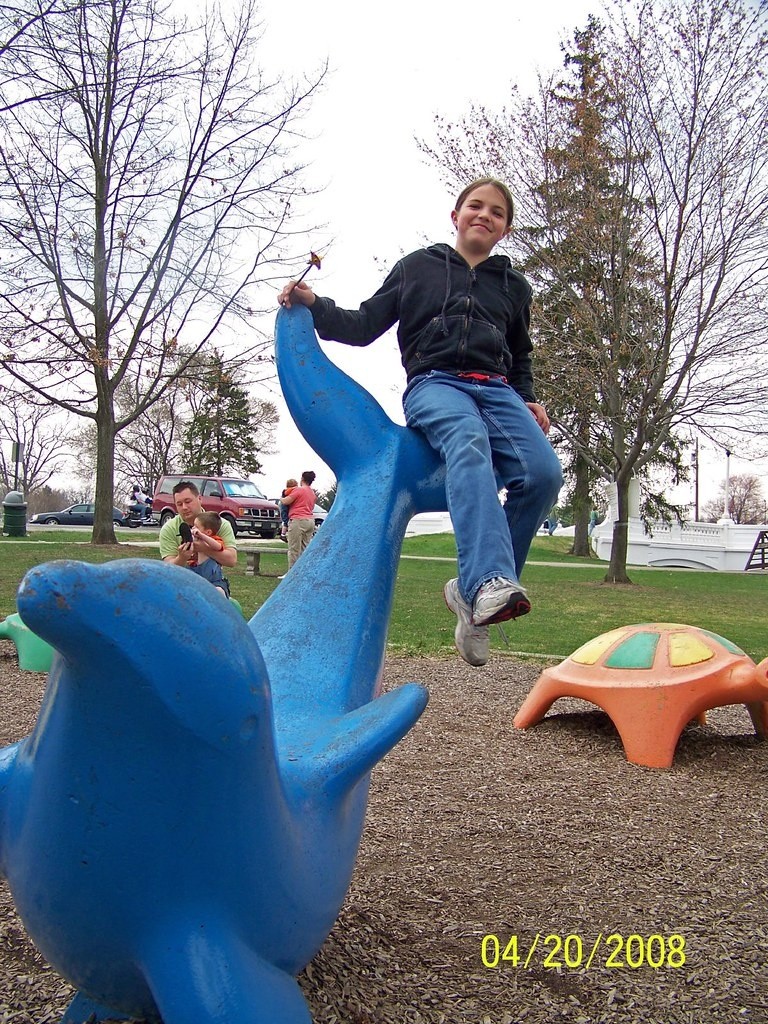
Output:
[
  {"left": 138, "top": 487, "right": 156, "bottom": 522},
  {"left": 186, "top": 510, "right": 230, "bottom": 601},
  {"left": 277, "top": 177, "right": 563, "bottom": 666},
  {"left": 280, "top": 479, "right": 300, "bottom": 544},
  {"left": 159, "top": 481, "right": 237, "bottom": 600},
  {"left": 547, "top": 503, "right": 558, "bottom": 536},
  {"left": 278, "top": 470, "right": 316, "bottom": 580},
  {"left": 128, "top": 485, "right": 147, "bottom": 520},
  {"left": 587, "top": 506, "right": 599, "bottom": 536}
]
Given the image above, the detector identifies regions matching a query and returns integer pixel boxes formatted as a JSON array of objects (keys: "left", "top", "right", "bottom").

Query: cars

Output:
[
  {"left": 30, "top": 503, "right": 128, "bottom": 528},
  {"left": 267, "top": 498, "right": 329, "bottom": 540}
]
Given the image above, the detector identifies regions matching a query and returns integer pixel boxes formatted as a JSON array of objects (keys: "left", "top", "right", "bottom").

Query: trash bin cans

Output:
[{"left": 2, "top": 491, "right": 28, "bottom": 536}]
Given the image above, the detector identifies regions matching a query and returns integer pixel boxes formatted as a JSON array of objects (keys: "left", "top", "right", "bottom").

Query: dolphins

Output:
[{"left": 0, "top": 296, "right": 508, "bottom": 1023}]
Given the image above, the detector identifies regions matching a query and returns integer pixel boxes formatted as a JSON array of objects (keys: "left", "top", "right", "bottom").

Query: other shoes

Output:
[
  {"left": 278, "top": 573, "right": 287, "bottom": 579},
  {"left": 176, "top": 523, "right": 193, "bottom": 550}
]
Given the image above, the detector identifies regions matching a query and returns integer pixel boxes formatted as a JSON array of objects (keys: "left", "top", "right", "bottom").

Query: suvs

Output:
[{"left": 151, "top": 475, "right": 283, "bottom": 539}]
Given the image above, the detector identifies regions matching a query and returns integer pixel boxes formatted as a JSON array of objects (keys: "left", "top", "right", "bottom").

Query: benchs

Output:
[{"left": 236, "top": 546, "right": 289, "bottom": 577}]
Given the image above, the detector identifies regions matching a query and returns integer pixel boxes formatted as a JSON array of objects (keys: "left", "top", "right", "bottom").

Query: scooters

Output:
[{"left": 124, "top": 499, "right": 159, "bottom": 528}]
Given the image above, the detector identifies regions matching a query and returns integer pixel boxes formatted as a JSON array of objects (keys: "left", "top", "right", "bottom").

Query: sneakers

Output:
[
  {"left": 472, "top": 577, "right": 531, "bottom": 625},
  {"left": 445, "top": 578, "right": 491, "bottom": 666}
]
